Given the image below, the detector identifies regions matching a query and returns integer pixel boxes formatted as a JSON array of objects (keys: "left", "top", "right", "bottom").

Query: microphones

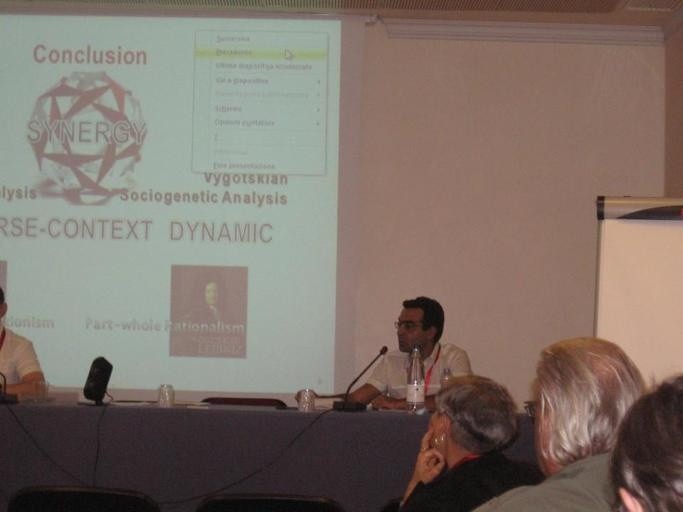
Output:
[{"left": 335, "top": 346, "right": 388, "bottom": 413}]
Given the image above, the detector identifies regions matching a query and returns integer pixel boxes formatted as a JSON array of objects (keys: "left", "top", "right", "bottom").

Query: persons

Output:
[
  {"left": 393, "top": 374, "right": 532, "bottom": 511},
  {"left": 294, "top": 295, "right": 474, "bottom": 412},
  {"left": 185, "top": 277, "right": 241, "bottom": 355},
  {"left": 470, "top": 337, "right": 648, "bottom": 511},
  {"left": 605, "top": 372, "right": 683, "bottom": 512},
  {"left": 0, "top": 285, "right": 45, "bottom": 399}
]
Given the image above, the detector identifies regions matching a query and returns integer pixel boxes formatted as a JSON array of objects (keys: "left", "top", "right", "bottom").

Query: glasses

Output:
[
  {"left": 522, "top": 399, "right": 546, "bottom": 418},
  {"left": 391, "top": 319, "right": 425, "bottom": 329}
]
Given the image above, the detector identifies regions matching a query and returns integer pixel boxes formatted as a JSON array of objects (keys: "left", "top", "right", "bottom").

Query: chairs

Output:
[
  {"left": 196, "top": 493, "right": 344, "bottom": 512},
  {"left": 6, "top": 485, "right": 160, "bottom": 512},
  {"left": 201, "top": 397, "right": 286, "bottom": 409}
]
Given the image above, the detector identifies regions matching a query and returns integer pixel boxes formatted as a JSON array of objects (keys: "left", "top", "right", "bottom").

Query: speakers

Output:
[{"left": 85, "top": 357, "right": 113, "bottom": 406}]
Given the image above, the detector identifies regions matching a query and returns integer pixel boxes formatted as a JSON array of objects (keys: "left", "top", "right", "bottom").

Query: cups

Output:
[
  {"left": 32, "top": 381, "right": 47, "bottom": 407},
  {"left": 159, "top": 384, "right": 175, "bottom": 410},
  {"left": 404, "top": 345, "right": 426, "bottom": 416},
  {"left": 296, "top": 388, "right": 314, "bottom": 414}
]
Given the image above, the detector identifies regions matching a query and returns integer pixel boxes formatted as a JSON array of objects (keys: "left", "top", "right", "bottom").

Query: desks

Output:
[{"left": 0, "top": 387, "right": 531, "bottom": 512}]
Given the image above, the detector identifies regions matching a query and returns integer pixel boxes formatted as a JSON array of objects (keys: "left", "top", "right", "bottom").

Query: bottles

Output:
[{"left": 439, "top": 367, "right": 453, "bottom": 388}]
